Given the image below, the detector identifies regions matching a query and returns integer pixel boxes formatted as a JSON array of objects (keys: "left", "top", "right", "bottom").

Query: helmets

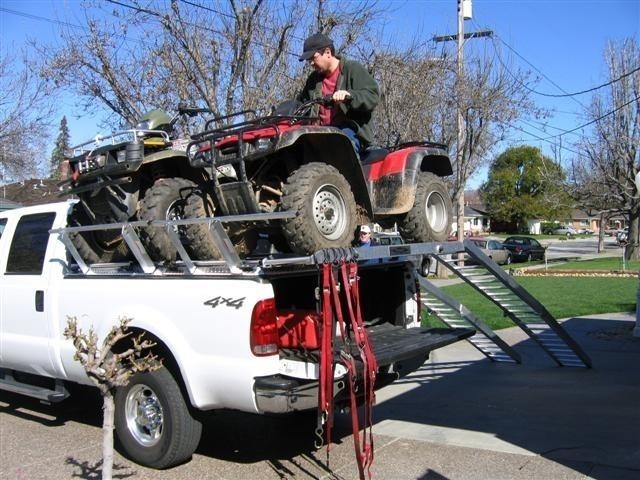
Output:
[{"left": 131, "top": 108, "right": 174, "bottom": 141}]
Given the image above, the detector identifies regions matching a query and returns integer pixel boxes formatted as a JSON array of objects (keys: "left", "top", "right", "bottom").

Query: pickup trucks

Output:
[{"left": 1, "top": 203, "right": 478, "bottom": 472}]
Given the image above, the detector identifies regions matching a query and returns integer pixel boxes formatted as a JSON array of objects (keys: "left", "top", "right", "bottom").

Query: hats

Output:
[
  {"left": 298, "top": 34, "right": 333, "bottom": 63},
  {"left": 359, "top": 225, "right": 370, "bottom": 234}
]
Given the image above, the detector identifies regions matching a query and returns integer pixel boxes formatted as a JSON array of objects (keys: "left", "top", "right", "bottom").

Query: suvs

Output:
[
  {"left": 180, "top": 91, "right": 457, "bottom": 260},
  {"left": 55, "top": 106, "right": 266, "bottom": 263},
  {"left": 372, "top": 232, "right": 432, "bottom": 276}
]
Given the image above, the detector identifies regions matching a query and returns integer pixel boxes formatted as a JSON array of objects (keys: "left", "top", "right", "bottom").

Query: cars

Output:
[
  {"left": 616, "top": 225, "right": 629, "bottom": 242},
  {"left": 500, "top": 236, "right": 546, "bottom": 263},
  {"left": 447, "top": 229, "right": 473, "bottom": 242},
  {"left": 577, "top": 227, "right": 595, "bottom": 235},
  {"left": 543, "top": 225, "right": 578, "bottom": 237},
  {"left": 452, "top": 236, "right": 513, "bottom": 266}
]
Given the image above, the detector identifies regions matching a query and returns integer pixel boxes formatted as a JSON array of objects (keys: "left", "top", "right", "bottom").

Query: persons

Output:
[
  {"left": 244, "top": 33, "right": 381, "bottom": 210},
  {"left": 358, "top": 225, "right": 378, "bottom": 246}
]
